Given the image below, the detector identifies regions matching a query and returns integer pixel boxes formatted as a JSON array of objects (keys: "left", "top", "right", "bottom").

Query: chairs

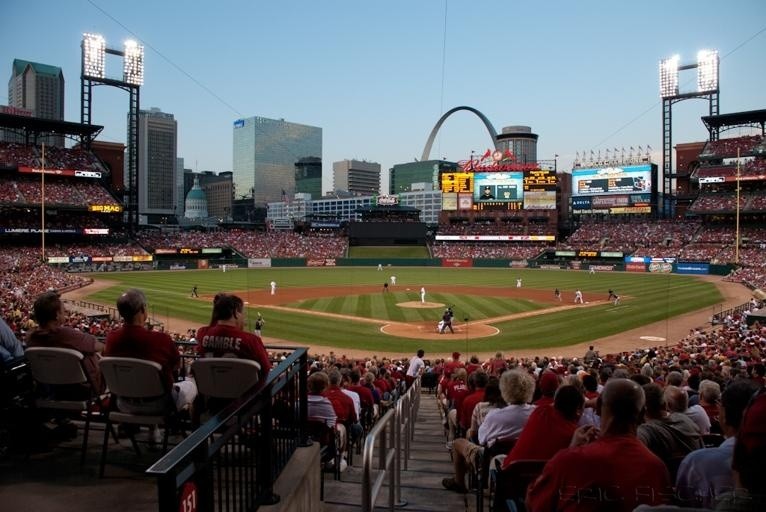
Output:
[
  {"left": 190, "top": 358, "right": 263, "bottom": 455},
  {"left": 21, "top": 347, "right": 119, "bottom": 454},
  {"left": 306, "top": 350, "right": 730, "bottom": 511},
  {"left": 99, "top": 356, "right": 186, "bottom": 463}
]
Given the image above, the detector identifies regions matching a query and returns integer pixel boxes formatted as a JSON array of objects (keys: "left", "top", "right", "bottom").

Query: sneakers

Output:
[{"left": 442, "top": 478, "right": 466, "bottom": 491}]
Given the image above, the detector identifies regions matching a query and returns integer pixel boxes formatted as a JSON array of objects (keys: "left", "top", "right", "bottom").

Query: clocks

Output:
[{"left": 492, "top": 149, "right": 504, "bottom": 161}]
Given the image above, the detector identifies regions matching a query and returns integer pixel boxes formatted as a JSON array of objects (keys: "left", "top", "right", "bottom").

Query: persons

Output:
[{"left": 2, "top": 133, "right": 766, "bottom": 510}]
